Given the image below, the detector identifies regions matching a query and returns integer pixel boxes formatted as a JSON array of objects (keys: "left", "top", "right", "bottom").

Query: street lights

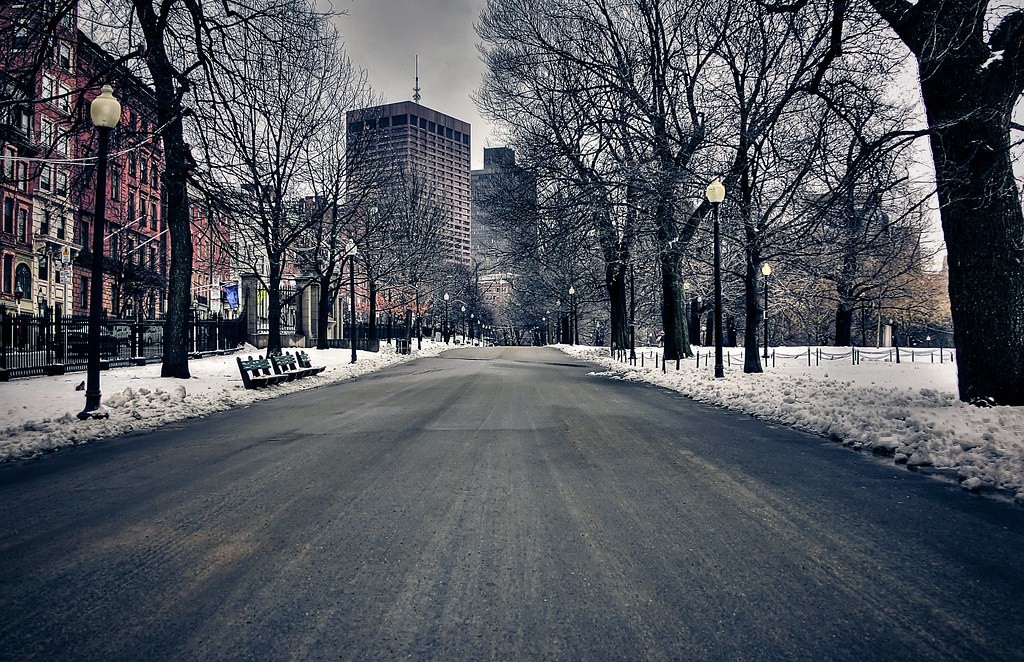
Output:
[
  {"left": 569, "top": 286, "right": 575, "bottom": 347},
  {"left": 860, "top": 292, "right": 865, "bottom": 346},
  {"left": 541, "top": 310, "right": 550, "bottom": 346},
  {"left": 461, "top": 305, "right": 466, "bottom": 343},
  {"left": 477, "top": 320, "right": 494, "bottom": 347},
  {"left": 470, "top": 313, "right": 474, "bottom": 345},
  {"left": 76, "top": 83, "right": 122, "bottom": 421},
  {"left": 345, "top": 238, "right": 357, "bottom": 364},
  {"left": 762, "top": 263, "right": 771, "bottom": 357},
  {"left": 444, "top": 292, "right": 449, "bottom": 345},
  {"left": 705, "top": 179, "right": 726, "bottom": 378},
  {"left": 555, "top": 298, "right": 561, "bottom": 344}
]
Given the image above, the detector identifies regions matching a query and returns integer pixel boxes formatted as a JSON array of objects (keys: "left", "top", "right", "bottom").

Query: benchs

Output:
[
  {"left": 236, "top": 355, "right": 288, "bottom": 389},
  {"left": 271, "top": 351, "right": 308, "bottom": 381},
  {"left": 295, "top": 351, "right": 326, "bottom": 376}
]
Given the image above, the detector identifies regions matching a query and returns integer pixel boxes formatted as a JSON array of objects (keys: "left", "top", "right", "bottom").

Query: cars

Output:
[
  {"left": 55, "top": 320, "right": 120, "bottom": 360},
  {"left": 61, "top": 322, "right": 164, "bottom": 345}
]
